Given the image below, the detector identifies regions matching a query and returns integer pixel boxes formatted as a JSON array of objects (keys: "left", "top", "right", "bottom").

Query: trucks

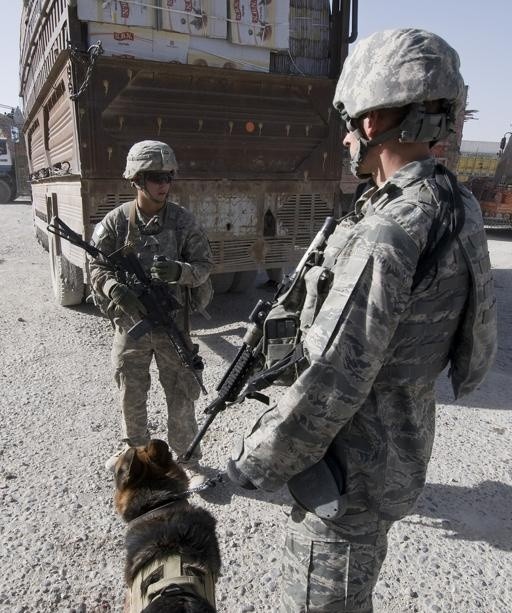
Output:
[
  {"left": 463, "top": 130, "right": 512, "bottom": 229},
  {"left": 17, "top": 0, "right": 361, "bottom": 311},
  {"left": 0, "top": 121, "right": 34, "bottom": 203}
]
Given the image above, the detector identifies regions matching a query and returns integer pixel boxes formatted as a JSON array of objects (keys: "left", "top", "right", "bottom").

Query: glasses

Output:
[{"left": 147, "top": 173, "right": 172, "bottom": 185}]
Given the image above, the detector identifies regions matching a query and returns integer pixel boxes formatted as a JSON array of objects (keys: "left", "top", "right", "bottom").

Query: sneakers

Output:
[{"left": 182, "top": 462, "right": 206, "bottom": 487}]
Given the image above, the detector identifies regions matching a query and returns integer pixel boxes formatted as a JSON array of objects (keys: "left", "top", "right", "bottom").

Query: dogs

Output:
[{"left": 103, "top": 436, "right": 222, "bottom": 613}]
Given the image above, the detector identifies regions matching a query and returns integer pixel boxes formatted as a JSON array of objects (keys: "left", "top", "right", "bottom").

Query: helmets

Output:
[
  {"left": 332, "top": 27, "right": 465, "bottom": 119},
  {"left": 123, "top": 139, "right": 178, "bottom": 180}
]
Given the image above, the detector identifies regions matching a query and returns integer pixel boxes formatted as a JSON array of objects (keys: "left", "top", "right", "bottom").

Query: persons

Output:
[
  {"left": 85, "top": 139, "right": 215, "bottom": 490},
  {"left": 188, "top": 25, "right": 498, "bottom": 612}
]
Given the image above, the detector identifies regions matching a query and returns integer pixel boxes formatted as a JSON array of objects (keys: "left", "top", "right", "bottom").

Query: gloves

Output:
[
  {"left": 110, "top": 282, "right": 146, "bottom": 326},
  {"left": 151, "top": 255, "right": 180, "bottom": 283}
]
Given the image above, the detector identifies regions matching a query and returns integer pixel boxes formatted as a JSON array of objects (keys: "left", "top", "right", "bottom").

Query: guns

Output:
[
  {"left": 108, "top": 245, "right": 208, "bottom": 396},
  {"left": 183, "top": 216, "right": 340, "bottom": 461}
]
